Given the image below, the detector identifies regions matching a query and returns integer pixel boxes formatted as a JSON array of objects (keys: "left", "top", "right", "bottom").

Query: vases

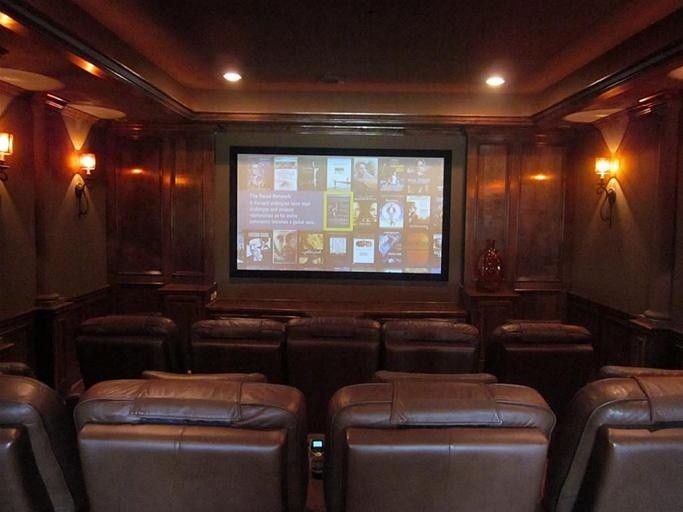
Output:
[{"left": 479, "top": 239, "right": 504, "bottom": 292}]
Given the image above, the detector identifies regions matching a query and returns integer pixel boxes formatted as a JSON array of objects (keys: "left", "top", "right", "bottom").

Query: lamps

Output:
[
  {"left": 594, "top": 157, "right": 615, "bottom": 230},
  {"left": 75, "top": 153, "right": 96, "bottom": 215},
  {"left": 0, "top": 131, "right": 14, "bottom": 182}
]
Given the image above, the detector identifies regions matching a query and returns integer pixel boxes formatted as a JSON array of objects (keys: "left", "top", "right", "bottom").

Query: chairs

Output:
[{"left": 0, "top": 315, "right": 683, "bottom": 512}]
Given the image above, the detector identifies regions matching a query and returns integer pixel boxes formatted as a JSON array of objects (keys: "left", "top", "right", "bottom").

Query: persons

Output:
[
  {"left": 366, "top": 200, "right": 376, "bottom": 226},
  {"left": 385, "top": 202, "right": 398, "bottom": 224},
  {"left": 242, "top": 234, "right": 271, "bottom": 264},
  {"left": 354, "top": 162, "right": 374, "bottom": 195},
  {"left": 271, "top": 230, "right": 298, "bottom": 265},
  {"left": 406, "top": 201, "right": 421, "bottom": 224},
  {"left": 352, "top": 201, "right": 362, "bottom": 228}
]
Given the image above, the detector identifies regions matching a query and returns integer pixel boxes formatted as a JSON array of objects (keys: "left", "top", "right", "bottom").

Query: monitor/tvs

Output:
[{"left": 228, "top": 145, "right": 452, "bottom": 282}]
